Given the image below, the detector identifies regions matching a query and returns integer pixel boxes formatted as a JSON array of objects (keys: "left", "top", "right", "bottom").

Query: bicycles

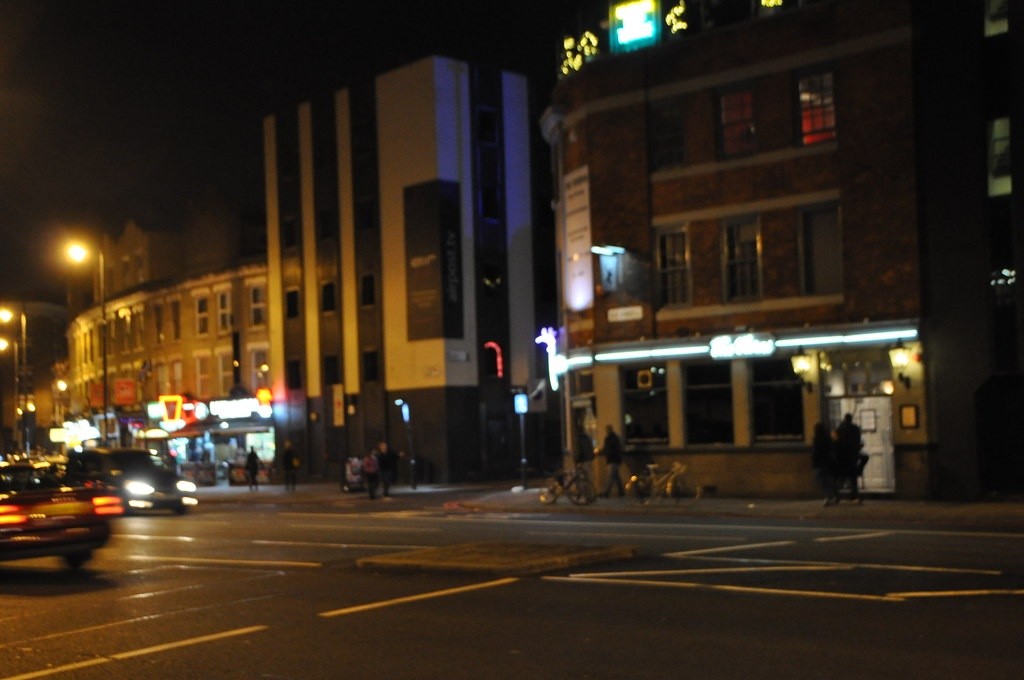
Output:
[{"left": 539, "top": 452, "right": 700, "bottom": 509}]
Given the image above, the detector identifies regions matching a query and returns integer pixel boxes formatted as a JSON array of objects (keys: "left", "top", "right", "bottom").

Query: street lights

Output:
[
  {"left": 69, "top": 243, "right": 107, "bottom": 447},
  {"left": 395, "top": 399, "right": 416, "bottom": 490},
  {"left": 0, "top": 310, "right": 26, "bottom": 459},
  {"left": 514, "top": 394, "right": 528, "bottom": 489}
]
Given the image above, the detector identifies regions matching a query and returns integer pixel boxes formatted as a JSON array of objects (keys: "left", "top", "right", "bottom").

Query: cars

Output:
[{"left": 0, "top": 447, "right": 198, "bottom": 568}]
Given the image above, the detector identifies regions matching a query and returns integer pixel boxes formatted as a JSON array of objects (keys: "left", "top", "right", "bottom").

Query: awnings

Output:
[{"left": 169, "top": 419, "right": 268, "bottom": 438}]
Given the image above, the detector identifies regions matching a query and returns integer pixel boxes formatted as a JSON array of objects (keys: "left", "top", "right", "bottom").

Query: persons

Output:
[
  {"left": 244, "top": 446, "right": 265, "bottom": 491},
  {"left": 363, "top": 441, "right": 392, "bottom": 499},
  {"left": 811, "top": 412, "right": 864, "bottom": 505},
  {"left": 574, "top": 426, "right": 595, "bottom": 499},
  {"left": 283, "top": 441, "right": 299, "bottom": 488},
  {"left": 599, "top": 424, "right": 625, "bottom": 498}
]
